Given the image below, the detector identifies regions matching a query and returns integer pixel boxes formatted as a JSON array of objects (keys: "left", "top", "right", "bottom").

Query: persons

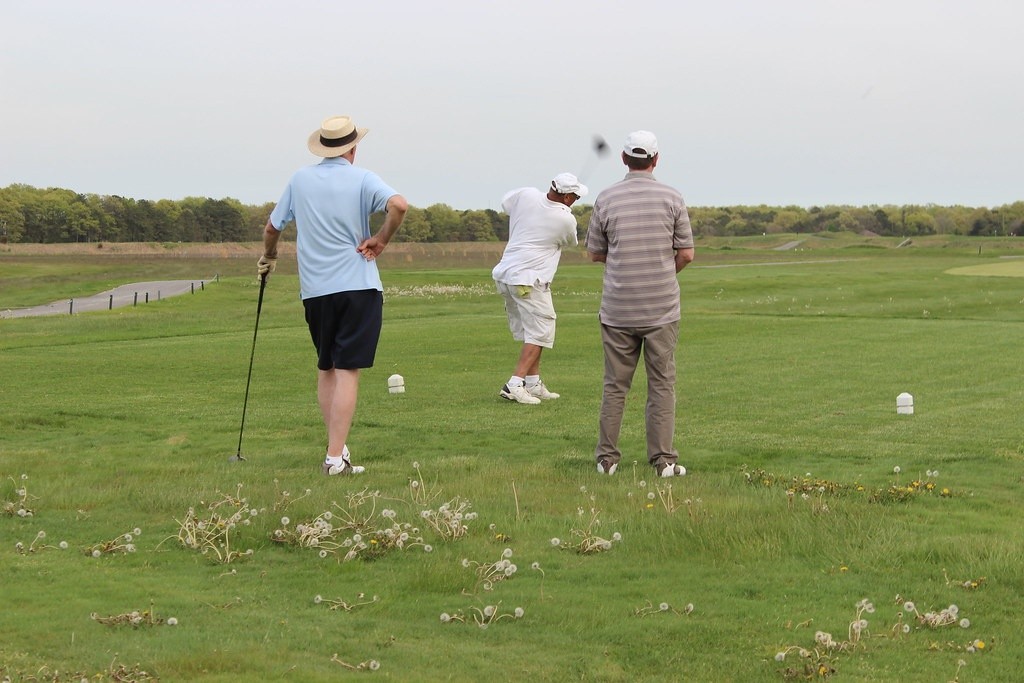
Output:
[
  {"left": 257, "top": 115, "right": 408, "bottom": 474},
  {"left": 492, "top": 173, "right": 588, "bottom": 405},
  {"left": 584, "top": 132, "right": 696, "bottom": 477}
]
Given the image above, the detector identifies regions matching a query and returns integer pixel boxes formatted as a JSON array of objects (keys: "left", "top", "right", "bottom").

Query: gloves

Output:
[{"left": 257, "top": 255, "right": 277, "bottom": 289}]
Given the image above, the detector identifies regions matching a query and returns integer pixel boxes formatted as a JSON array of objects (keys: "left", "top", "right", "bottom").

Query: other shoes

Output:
[
  {"left": 655, "top": 463, "right": 687, "bottom": 479},
  {"left": 596, "top": 459, "right": 618, "bottom": 476}
]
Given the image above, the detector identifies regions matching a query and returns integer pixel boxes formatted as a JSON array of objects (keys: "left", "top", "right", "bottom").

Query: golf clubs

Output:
[{"left": 227, "top": 263, "right": 269, "bottom": 464}]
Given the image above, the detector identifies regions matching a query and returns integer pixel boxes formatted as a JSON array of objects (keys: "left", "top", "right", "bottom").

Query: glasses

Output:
[{"left": 561, "top": 193, "right": 580, "bottom": 200}]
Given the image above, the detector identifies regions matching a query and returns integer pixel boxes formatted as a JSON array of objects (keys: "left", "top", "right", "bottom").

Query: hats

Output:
[
  {"left": 623, "top": 130, "right": 658, "bottom": 158},
  {"left": 308, "top": 114, "right": 369, "bottom": 158},
  {"left": 551, "top": 173, "right": 588, "bottom": 197}
]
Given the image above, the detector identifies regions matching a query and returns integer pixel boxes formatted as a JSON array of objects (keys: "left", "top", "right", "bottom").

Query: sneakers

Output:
[
  {"left": 525, "top": 380, "right": 560, "bottom": 400},
  {"left": 326, "top": 444, "right": 351, "bottom": 464},
  {"left": 499, "top": 381, "right": 541, "bottom": 404},
  {"left": 322, "top": 455, "right": 365, "bottom": 476}
]
